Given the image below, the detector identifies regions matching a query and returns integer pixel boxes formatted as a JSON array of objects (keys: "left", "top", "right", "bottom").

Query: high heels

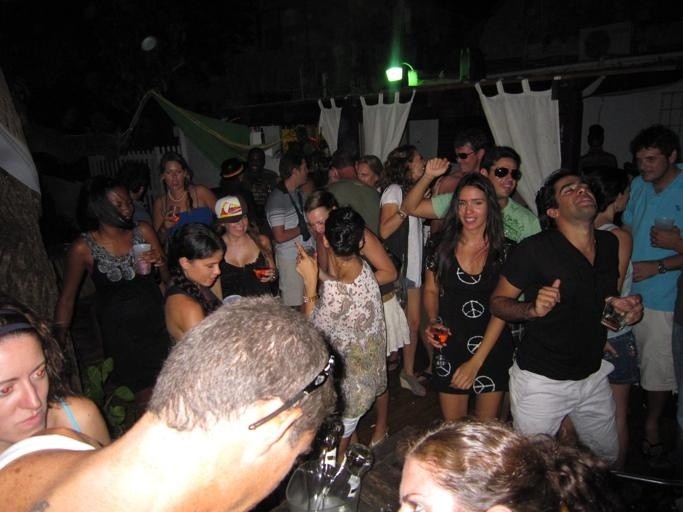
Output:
[{"left": 398, "top": 368, "right": 427, "bottom": 397}]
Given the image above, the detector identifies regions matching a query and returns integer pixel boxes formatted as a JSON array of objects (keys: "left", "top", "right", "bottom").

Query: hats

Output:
[
  {"left": 213, "top": 194, "right": 249, "bottom": 225},
  {"left": 219, "top": 156, "right": 245, "bottom": 179}
]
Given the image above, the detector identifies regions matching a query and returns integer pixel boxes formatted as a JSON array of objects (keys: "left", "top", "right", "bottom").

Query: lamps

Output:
[{"left": 385, "top": 61, "right": 419, "bottom": 87}]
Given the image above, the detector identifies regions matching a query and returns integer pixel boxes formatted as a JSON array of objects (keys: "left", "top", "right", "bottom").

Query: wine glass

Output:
[
  {"left": 168, "top": 205, "right": 181, "bottom": 222},
  {"left": 430, "top": 315, "right": 450, "bottom": 366},
  {"left": 252, "top": 258, "right": 274, "bottom": 295}
]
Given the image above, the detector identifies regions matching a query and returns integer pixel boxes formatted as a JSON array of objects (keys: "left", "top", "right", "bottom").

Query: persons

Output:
[
  {"left": 0, "top": 298, "right": 111, "bottom": 458},
  {"left": 390, "top": 417, "right": 609, "bottom": 511},
  {"left": 0, "top": 293, "right": 339, "bottom": 512},
  {"left": 50, "top": 123, "right": 681, "bottom": 465}
]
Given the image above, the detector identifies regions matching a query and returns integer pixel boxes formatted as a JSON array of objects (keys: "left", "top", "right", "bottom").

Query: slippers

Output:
[
  {"left": 413, "top": 369, "right": 432, "bottom": 384},
  {"left": 368, "top": 432, "right": 390, "bottom": 449},
  {"left": 646, "top": 452, "right": 679, "bottom": 469},
  {"left": 641, "top": 422, "right": 664, "bottom": 463}
]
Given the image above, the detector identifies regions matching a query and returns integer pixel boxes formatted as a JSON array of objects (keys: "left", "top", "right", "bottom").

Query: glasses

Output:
[
  {"left": 452, "top": 150, "right": 476, "bottom": 159},
  {"left": 489, "top": 167, "right": 522, "bottom": 180}
]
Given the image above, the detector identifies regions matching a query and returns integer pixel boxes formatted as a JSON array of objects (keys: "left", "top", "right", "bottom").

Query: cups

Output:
[
  {"left": 316, "top": 423, "right": 345, "bottom": 476},
  {"left": 286, "top": 461, "right": 358, "bottom": 511},
  {"left": 327, "top": 443, "right": 373, "bottom": 511},
  {"left": 601, "top": 296, "right": 626, "bottom": 330},
  {"left": 133, "top": 243, "right": 151, "bottom": 274}
]
